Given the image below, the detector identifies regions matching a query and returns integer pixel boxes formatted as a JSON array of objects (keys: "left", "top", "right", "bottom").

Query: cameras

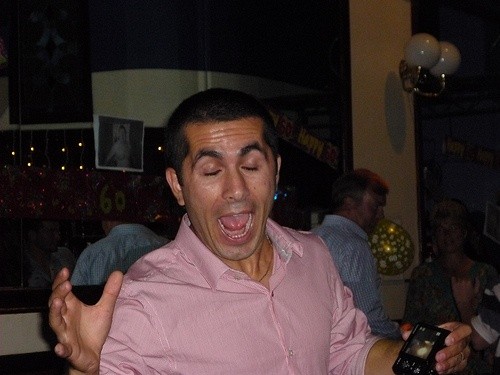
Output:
[{"left": 392, "top": 322, "right": 451, "bottom": 375}]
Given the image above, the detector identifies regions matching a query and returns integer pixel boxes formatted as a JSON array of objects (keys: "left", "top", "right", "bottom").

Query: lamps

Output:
[{"left": 399, "top": 33, "right": 460, "bottom": 97}]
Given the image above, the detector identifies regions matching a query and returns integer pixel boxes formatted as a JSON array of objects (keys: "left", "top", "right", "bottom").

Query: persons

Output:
[
  {"left": 105, "top": 125, "right": 137, "bottom": 168},
  {"left": 403, "top": 199, "right": 500, "bottom": 331},
  {"left": 47, "top": 87, "right": 473, "bottom": 374},
  {"left": 308, "top": 167, "right": 407, "bottom": 332},
  {"left": 79, "top": 201, "right": 176, "bottom": 285},
  {"left": 450, "top": 265, "right": 500, "bottom": 374}
]
